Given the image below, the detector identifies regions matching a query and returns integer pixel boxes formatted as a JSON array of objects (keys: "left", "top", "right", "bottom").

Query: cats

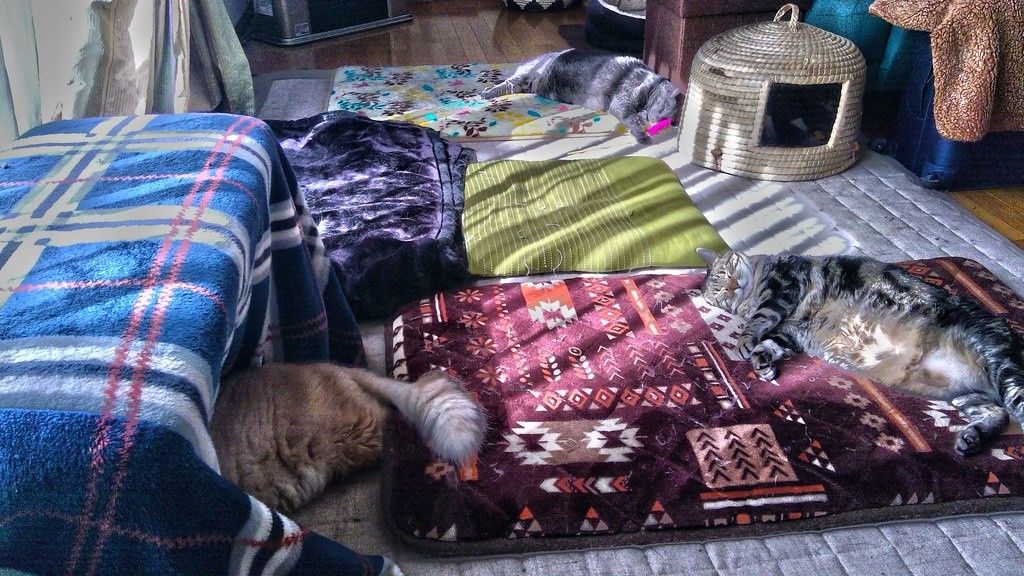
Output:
[
  {"left": 207, "top": 364, "right": 489, "bottom": 513},
  {"left": 481, "top": 48, "right": 681, "bottom": 146},
  {"left": 682, "top": 246, "right": 1023, "bottom": 457}
]
